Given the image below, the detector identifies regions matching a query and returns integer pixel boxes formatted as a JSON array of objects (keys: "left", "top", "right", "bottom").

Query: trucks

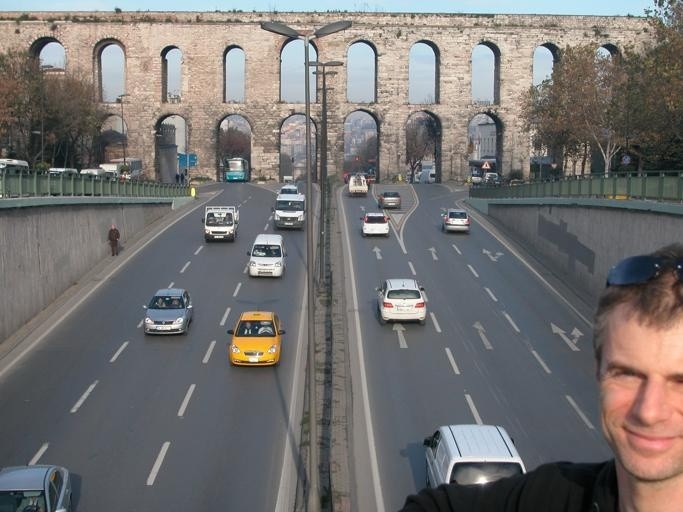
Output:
[
  {"left": 270, "top": 193, "right": 308, "bottom": 231},
  {"left": 276, "top": 182, "right": 300, "bottom": 195},
  {"left": 346, "top": 170, "right": 376, "bottom": 195},
  {"left": 281, "top": 175, "right": 296, "bottom": 184},
  {"left": 202, "top": 204, "right": 241, "bottom": 241}
]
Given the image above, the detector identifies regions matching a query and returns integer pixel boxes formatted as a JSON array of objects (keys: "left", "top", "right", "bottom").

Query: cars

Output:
[
  {"left": 0, "top": 461, "right": 72, "bottom": 512},
  {"left": 439, "top": 207, "right": 472, "bottom": 234},
  {"left": 375, "top": 190, "right": 402, "bottom": 210},
  {"left": 142, "top": 287, "right": 195, "bottom": 335},
  {"left": 225, "top": 310, "right": 287, "bottom": 368},
  {"left": 372, "top": 278, "right": 431, "bottom": 324},
  {"left": 0, "top": 154, "right": 144, "bottom": 185},
  {"left": 469, "top": 163, "right": 499, "bottom": 186},
  {"left": 408, "top": 158, "right": 437, "bottom": 184},
  {"left": 359, "top": 212, "right": 391, "bottom": 237}
]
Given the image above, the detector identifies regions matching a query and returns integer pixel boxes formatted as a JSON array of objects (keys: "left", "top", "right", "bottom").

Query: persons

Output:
[
  {"left": 107, "top": 224, "right": 121, "bottom": 256},
  {"left": 394, "top": 242, "right": 682, "bottom": 511}
]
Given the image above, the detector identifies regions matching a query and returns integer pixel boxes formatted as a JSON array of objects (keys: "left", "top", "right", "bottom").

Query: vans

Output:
[
  {"left": 246, "top": 232, "right": 287, "bottom": 280},
  {"left": 419, "top": 420, "right": 527, "bottom": 492}
]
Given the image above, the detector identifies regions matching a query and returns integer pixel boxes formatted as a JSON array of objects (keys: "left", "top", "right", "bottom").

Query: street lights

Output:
[
  {"left": 258, "top": 17, "right": 349, "bottom": 512},
  {"left": 38, "top": 64, "right": 54, "bottom": 165},
  {"left": 297, "top": 58, "right": 344, "bottom": 209},
  {"left": 117, "top": 91, "right": 134, "bottom": 167}
]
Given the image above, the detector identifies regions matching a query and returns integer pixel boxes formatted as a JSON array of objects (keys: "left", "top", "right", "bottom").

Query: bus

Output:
[{"left": 224, "top": 157, "right": 250, "bottom": 183}]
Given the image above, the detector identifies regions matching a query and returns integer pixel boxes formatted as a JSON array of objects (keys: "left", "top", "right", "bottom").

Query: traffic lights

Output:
[{"left": 354, "top": 155, "right": 361, "bottom": 163}]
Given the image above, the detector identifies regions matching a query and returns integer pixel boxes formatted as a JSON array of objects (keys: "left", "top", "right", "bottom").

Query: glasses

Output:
[{"left": 602, "top": 252, "right": 681, "bottom": 289}]
